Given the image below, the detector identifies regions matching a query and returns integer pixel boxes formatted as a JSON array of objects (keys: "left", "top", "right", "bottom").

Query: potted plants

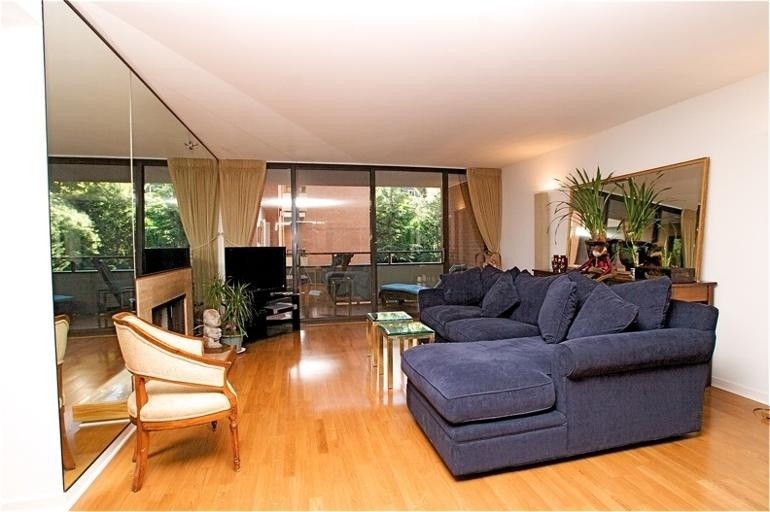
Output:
[
  {"left": 204, "top": 270, "right": 261, "bottom": 354},
  {"left": 544, "top": 164, "right": 617, "bottom": 268},
  {"left": 614, "top": 170, "right": 686, "bottom": 273}
]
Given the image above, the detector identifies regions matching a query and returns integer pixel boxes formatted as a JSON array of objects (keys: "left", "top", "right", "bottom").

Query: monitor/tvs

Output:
[
  {"left": 142, "top": 248, "right": 190, "bottom": 273},
  {"left": 224, "top": 246, "right": 287, "bottom": 292}
]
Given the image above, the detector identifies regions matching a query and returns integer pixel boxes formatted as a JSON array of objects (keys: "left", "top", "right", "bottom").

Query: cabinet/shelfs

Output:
[
  {"left": 242, "top": 292, "right": 301, "bottom": 346},
  {"left": 328, "top": 275, "right": 352, "bottom": 316}
]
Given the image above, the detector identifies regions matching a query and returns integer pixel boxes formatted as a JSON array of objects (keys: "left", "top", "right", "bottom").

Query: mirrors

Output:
[
  {"left": 567, "top": 156, "right": 710, "bottom": 282},
  {"left": 41, "top": 0, "right": 220, "bottom": 491}
]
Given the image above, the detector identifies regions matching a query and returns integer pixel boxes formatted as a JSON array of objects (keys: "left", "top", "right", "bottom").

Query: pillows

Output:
[{"left": 440, "top": 266, "right": 673, "bottom": 345}]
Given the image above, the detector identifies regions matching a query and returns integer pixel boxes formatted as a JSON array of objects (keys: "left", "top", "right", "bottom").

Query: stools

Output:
[{"left": 366, "top": 310, "right": 436, "bottom": 390}]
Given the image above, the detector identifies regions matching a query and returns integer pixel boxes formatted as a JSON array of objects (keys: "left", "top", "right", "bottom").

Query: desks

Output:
[{"left": 531, "top": 268, "right": 718, "bottom": 388}]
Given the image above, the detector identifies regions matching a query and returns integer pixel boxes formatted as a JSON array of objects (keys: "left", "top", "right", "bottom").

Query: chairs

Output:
[
  {"left": 53, "top": 294, "right": 74, "bottom": 322},
  {"left": 96, "top": 259, "right": 136, "bottom": 329},
  {"left": 54, "top": 313, "right": 75, "bottom": 470},
  {"left": 111, "top": 310, "right": 240, "bottom": 493},
  {"left": 379, "top": 264, "right": 466, "bottom": 320}
]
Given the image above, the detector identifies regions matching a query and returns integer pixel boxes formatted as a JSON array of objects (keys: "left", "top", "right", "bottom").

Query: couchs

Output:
[{"left": 400, "top": 263, "right": 720, "bottom": 482}]
